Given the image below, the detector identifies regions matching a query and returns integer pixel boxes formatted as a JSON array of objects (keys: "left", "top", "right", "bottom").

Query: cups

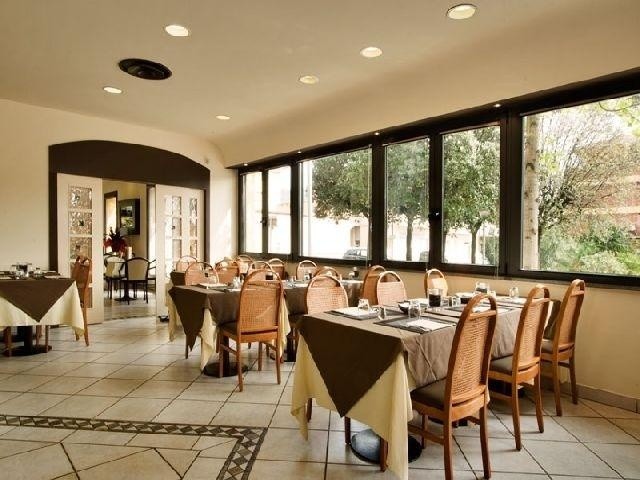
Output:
[
  {"left": 356, "top": 297, "right": 463, "bottom": 321},
  {"left": 487, "top": 287, "right": 520, "bottom": 300},
  {"left": 232, "top": 271, "right": 356, "bottom": 290}
]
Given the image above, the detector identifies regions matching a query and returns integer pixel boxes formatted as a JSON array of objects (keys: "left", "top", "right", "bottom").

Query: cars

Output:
[{"left": 344, "top": 248, "right": 369, "bottom": 261}]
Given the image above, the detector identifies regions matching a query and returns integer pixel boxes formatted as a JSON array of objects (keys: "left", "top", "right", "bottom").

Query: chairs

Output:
[{"left": 0, "top": 249, "right": 156, "bottom": 357}]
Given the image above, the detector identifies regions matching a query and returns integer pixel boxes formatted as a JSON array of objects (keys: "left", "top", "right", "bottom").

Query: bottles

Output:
[
  {"left": 475, "top": 281, "right": 489, "bottom": 293},
  {"left": 428, "top": 289, "right": 440, "bottom": 306},
  {"left": 34, "top": 268, "right": 43, "bottom": 280},
  {"left": 264, "top": 266, "right": 359, "bottom": 280}
]
